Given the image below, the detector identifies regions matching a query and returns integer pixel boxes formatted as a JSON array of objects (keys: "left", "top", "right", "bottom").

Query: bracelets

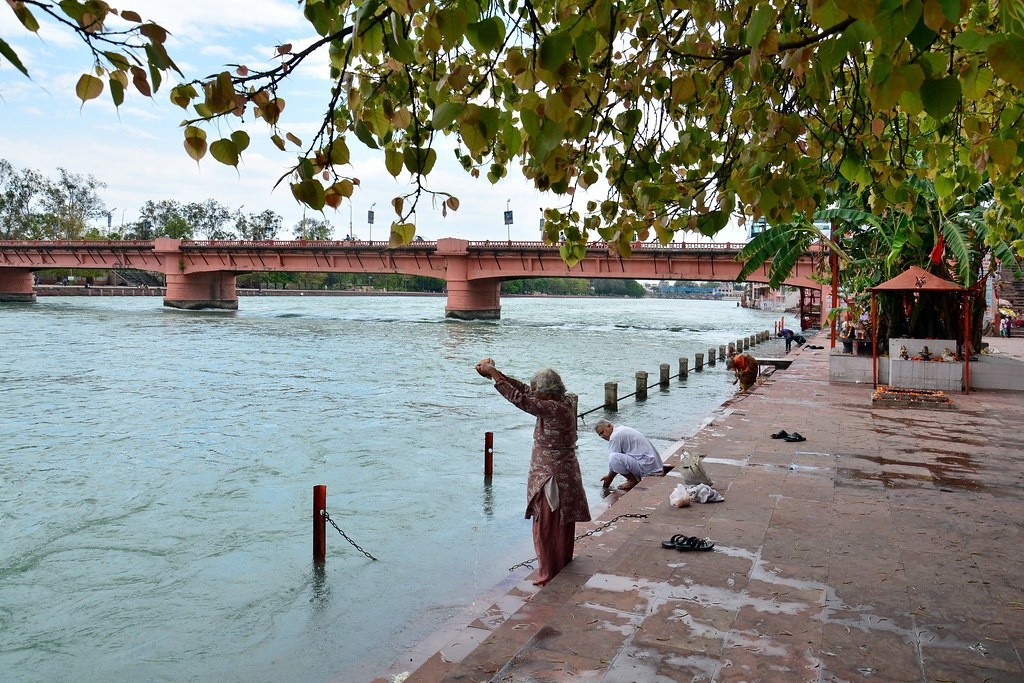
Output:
[{"left": 496, "top": 377, "right": 506, "bottom": 383}]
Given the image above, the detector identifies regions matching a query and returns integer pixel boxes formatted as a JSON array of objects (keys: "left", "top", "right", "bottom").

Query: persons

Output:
[
  {"left": 672, "top": 239, "right": 676, "bottom": 248},
  {"left": 999, "top": 314, "right": 1008, "bottom": 338},
  {"left": 559, "top": 231, "right": 567, "bottom": 247},
  {"left": 594, "top": 418, "right": 665, "bottom": 491},
  {"left": 33, "top": 230, "right": 425, "bottom": 246},
  {"left": 1005, "top": 316, "right": 1012, "bottom": 338},
  {"left": 985, "top": 305, "right": 991, "bottom": 317},
  {"left": 480, "top": 361, "right": 592, "bottom": 587},
  {"left": 787, "top": 331, "right": 806, "bottom": 347},
  {"left": 726, "top": 345, "right": 758, "bottom": 395},
  {"left": 1015, "top": 309, "right": 1024, "bottom": 328},
  {"left": 777, "top": 328, "right": 794, "bottom": 353}
]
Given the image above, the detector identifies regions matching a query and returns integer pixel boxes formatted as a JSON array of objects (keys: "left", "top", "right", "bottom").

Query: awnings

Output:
[{"left": 864, "top": 264, "right": 978, "bottom": 394}]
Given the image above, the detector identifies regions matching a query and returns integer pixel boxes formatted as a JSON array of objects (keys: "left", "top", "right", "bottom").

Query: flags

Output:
[{"left": 929, "top": 232, "right": 944, "bottom": 265}]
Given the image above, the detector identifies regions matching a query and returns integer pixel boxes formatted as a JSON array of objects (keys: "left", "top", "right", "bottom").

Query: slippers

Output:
[
  {"left": 676, "top": 536, "right": 714, "bottom": 551},
  {"left": 784, "top": 432, "right": 806, "bottom": 442},
  {"left": 662, "top": 534, "right": 689, "bottom": 549},
  {"left": 770, "top": 430, "right": 790, "bottom": 439}
]
